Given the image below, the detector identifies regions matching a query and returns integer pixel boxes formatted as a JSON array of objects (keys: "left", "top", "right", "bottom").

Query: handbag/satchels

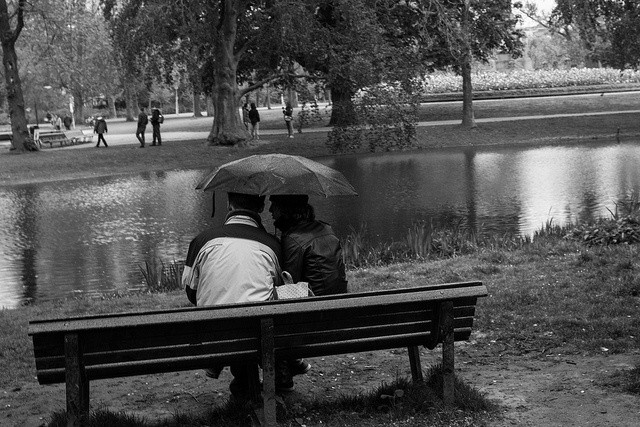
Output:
[
  {"left": 284, "top": 116, "right": 292, "bottom": 122},
  {"left": 274, "top": 271, "right": 309, "bottom": 300}
]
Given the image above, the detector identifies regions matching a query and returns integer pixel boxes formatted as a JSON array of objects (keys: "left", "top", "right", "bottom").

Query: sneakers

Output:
[
  {"left": 260, "top": 379, "right": 295, "bottom": 391},
  {"left": 294, "top": 358, "right": 311, "bottom": 375},
  {"left": 202, "top": 366, "right": 224, "bottom": 379}
]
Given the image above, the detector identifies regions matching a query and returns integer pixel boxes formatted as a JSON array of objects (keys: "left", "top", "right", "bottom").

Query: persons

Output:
[
  {"left": 247, "top": 101, "right": 262, "bottom": 140},
  {"left": 52, "top": 114, "right": 62, "bottom": 130},
  {"left": 88, "top": 116, "right": 94, "bottom": 129},
  {"left": 63, "top": 113, "right": 73, "bottom": 130},
  {"left": 241, "top": 101, "right": 249, "bottom": 133},
  {"left": 179, "top": 192, "right": 281, "bottom": 408},
  {"left": 135, "top": 106, "right": 149, "bottom": 148},
  {"left": 149, "top": 104, "right": 163, "bottom": 147},
  {"left": 281, "top": 100, "right": 296, "bottom": 140},
  {"left": 92, "top": 113, "right": 108, "bottom": 147},
  {"left": 266, "top": 194, "right": 349, "bottom": 390}
]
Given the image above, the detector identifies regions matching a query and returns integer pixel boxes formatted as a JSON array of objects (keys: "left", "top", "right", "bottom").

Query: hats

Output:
[{"left": 152, "top": 106, "right": 159, "bottom": 109}]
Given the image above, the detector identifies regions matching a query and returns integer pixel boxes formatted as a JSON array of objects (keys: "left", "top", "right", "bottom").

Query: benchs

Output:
[
  {"left": 27, "top": 279, "right": 489, "bottom": 424},
  {"left": 38, "top": 133, "right": 69, "bottom": 148}
]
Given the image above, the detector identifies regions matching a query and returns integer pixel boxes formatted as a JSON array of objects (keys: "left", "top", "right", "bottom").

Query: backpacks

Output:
[{"left": 158, "top": 114, "right": 164, "bottom": 123}]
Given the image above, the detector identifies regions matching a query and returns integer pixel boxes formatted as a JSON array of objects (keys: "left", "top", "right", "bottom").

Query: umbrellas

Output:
[{"left": 195, "top": 152, "right": 360, "bottom": 236}]
